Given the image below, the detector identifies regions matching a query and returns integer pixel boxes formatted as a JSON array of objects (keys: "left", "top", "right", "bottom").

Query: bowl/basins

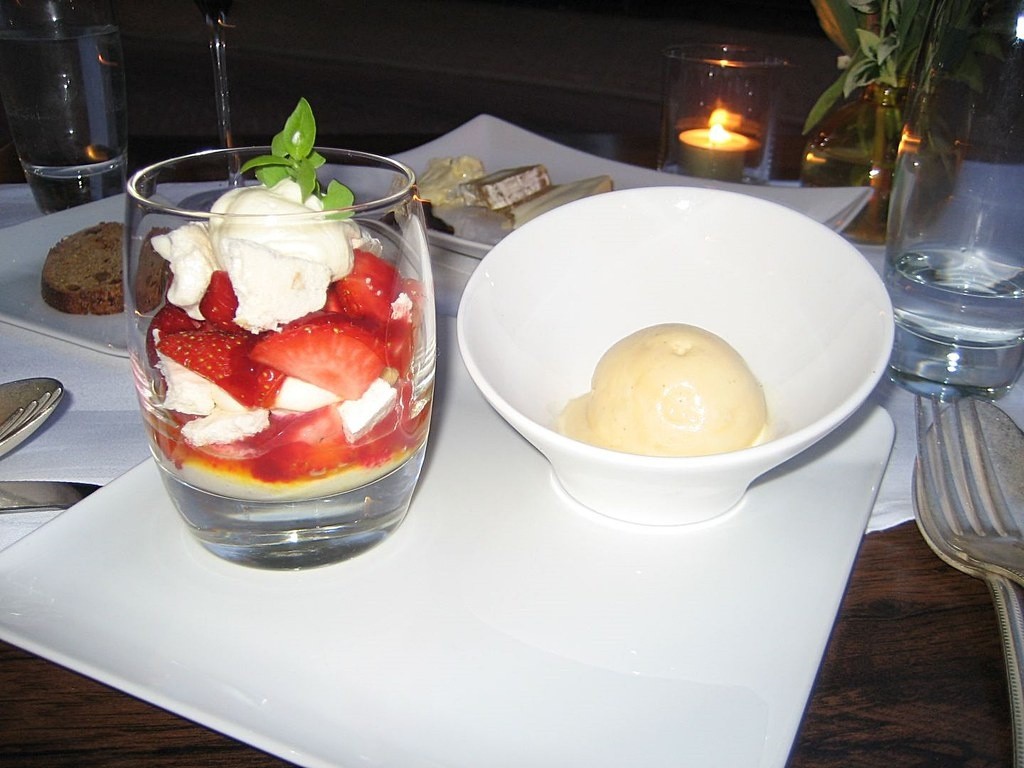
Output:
[{"left": 456, "top": 187, "right": 895, "bottom": 526}]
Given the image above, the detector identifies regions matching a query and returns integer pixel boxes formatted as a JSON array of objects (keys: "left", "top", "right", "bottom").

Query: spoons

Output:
[{"left": 911, "top": 396, "right": 1024, "bottom": 768}]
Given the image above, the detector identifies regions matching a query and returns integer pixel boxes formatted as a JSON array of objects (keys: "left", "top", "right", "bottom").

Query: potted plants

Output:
[{"left": 803, "top": 0, "right": 1024, "bottom": 243}]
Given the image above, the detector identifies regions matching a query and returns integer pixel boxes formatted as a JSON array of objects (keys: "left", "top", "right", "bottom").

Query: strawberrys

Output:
[{"left": 145, "top": 247, "right": 435, "bottom": 486}]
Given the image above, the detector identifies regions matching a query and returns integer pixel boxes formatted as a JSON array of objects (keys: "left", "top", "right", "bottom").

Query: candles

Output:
[{"left": 675, "top": 115, "right": 762, "bottom": 178}]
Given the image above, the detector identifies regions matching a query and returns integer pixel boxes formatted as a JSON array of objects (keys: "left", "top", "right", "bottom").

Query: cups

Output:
[
  {"left": 124, "top": 146, "right": 437, "bottom": 571},
  {"left": 654, "top": 43, "right": 792, "bottom": 185},
  {"left": 0, "top": 0, "right": 128, "bottom": 217},
  {"left": 882, "top": 0, "right": 1024, "bottom": 405}
]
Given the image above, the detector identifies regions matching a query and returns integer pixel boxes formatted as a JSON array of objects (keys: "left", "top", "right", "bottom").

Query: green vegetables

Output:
[{"left": 238, "top": 96, "right": 355, "bottom": 220}]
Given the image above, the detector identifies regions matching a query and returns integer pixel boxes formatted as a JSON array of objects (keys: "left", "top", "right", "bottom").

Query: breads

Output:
[
  {"left": 41, "top": 221, "right": 173, "bottom": 315},
  {"left": 459, "top": 164, "right": 613, "bottom": 232}
]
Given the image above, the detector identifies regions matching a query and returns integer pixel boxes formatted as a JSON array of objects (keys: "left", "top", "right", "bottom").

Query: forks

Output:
[
  {"left": 916, "top": 392, "right": 1024, "bottom": 591},
  {"left": 0, "top": 388, "right": 62, "bottom": 443}
]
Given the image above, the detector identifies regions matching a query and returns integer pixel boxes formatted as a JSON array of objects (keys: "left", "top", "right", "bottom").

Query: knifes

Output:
[{"left": 0, "top": 480, "right": 103, "bottom": 513}]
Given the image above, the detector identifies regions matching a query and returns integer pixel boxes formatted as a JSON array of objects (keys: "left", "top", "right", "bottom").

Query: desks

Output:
[{"left": 0, "top": 521, "right": 1024, "bottom": 768}]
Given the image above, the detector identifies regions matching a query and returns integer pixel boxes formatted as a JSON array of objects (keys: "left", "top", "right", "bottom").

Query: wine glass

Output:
[{"left": 170, "top": 0, "right": 259, "bottom": 221}]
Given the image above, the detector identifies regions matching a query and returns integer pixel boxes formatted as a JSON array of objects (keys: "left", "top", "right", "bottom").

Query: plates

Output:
[
  {"left": 0, "top": 377, "right": 64, "bottom": 458},
  {"left": 1, "top": 190, "right": 196, "bottom": 359},
  {"left": 2, "top": 317, "right": 895, "bottom": 767},
  {"left": 310, "top": 113, "right": 876, "bottom": 260}
]
